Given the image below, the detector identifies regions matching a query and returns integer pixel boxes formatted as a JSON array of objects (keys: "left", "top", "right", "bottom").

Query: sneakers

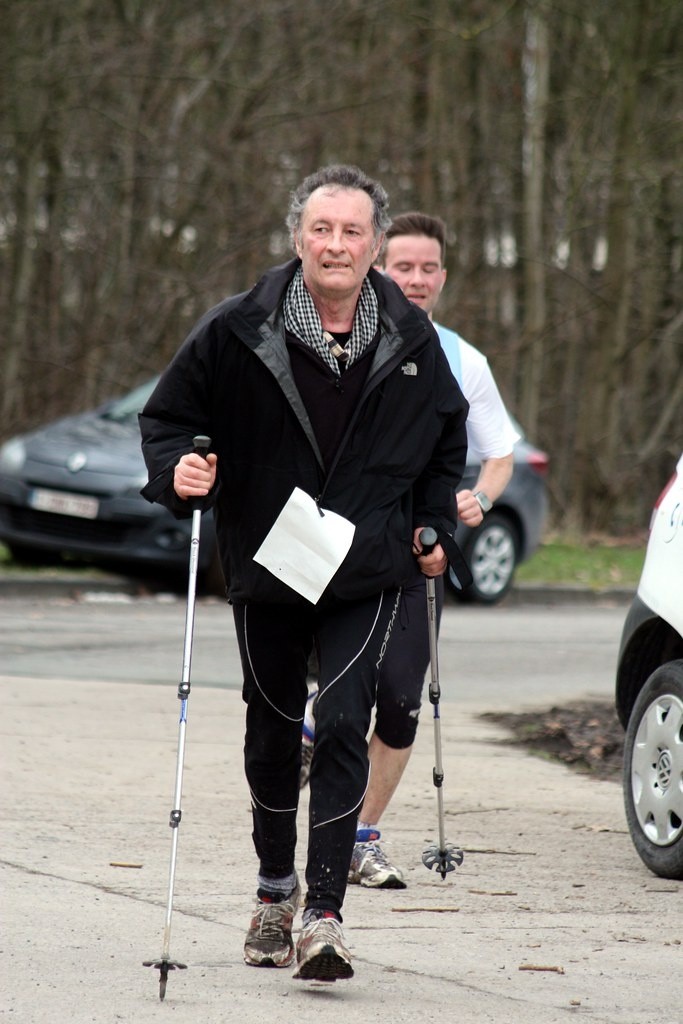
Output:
[
  {"left": 299, "top": 690, "right": 319, "bottom": 788},
  {"left": 347, "top": 828, "right": 408, "bottom": 888},
  {"left": 291, "top": 909, "right": 355, "bottom": 982},
  {"left": 242, "top": 879, "right": 302, "bottom": 968}
]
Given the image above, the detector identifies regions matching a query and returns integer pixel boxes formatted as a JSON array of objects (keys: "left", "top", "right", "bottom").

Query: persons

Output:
[
  {"left": 137, "top": 162, "right": 470, "bottom": 982},
  {"left": 300, "top": 211, "right": 521, "bottom": 888}
]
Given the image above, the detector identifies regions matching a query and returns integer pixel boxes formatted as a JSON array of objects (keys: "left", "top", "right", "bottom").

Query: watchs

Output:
[{"left": 473, "top": 491, "right": 493, "bottom": 516}]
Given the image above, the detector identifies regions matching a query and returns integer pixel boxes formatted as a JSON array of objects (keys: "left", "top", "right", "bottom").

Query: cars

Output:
[
  {"left": 613, "top": 449, "right": 683, "bottom": 882},
  {"left": 0, "top": 368, "right": 552, "bottom": 604}
]
